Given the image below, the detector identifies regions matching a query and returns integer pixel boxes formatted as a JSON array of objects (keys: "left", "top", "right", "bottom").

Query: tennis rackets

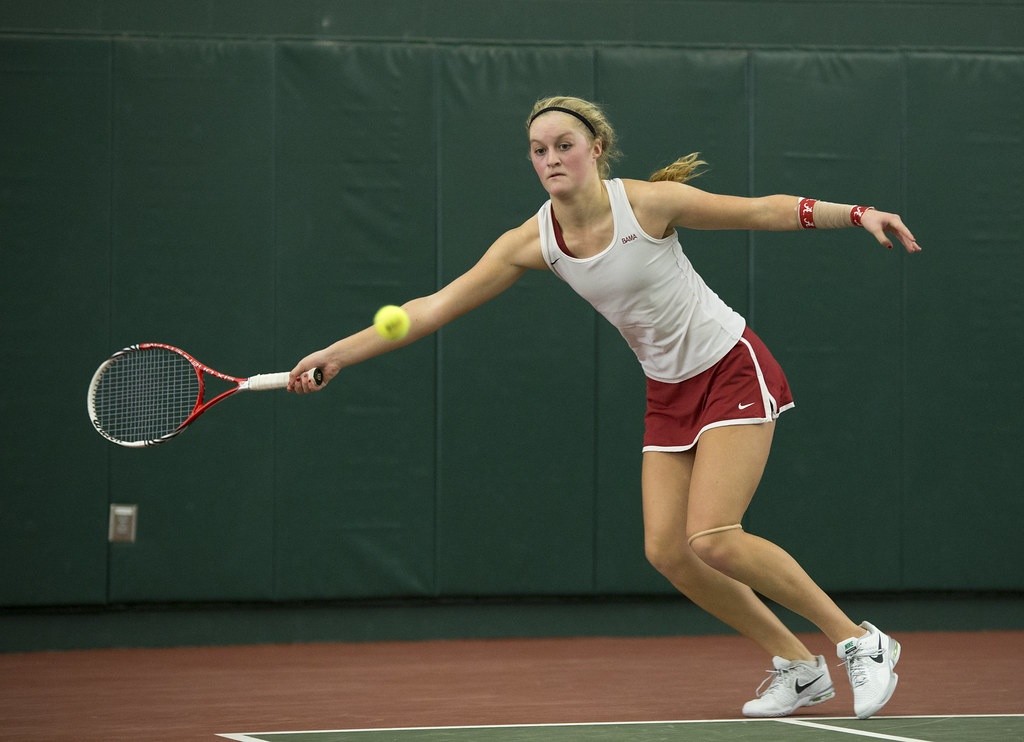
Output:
[{"left": 86, "top": 342, "right": 324, "bottom": 448}]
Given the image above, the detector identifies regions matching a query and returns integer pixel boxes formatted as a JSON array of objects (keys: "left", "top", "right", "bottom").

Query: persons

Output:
[{"left": 287, "top": 97, "right": 921, "bottom": 718}]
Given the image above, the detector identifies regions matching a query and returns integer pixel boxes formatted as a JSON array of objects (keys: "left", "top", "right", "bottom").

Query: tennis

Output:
[{"left": 373, "top": 304, "right": 410, "bottom": 342}]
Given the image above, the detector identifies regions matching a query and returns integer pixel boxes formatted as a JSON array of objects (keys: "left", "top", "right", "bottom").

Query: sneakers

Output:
[
  {"left": 837, "top": 621, "right": 901, "bottom": 718},
  {"left": 742, "top": 654, "right": 836, "bottom": 717}
]
